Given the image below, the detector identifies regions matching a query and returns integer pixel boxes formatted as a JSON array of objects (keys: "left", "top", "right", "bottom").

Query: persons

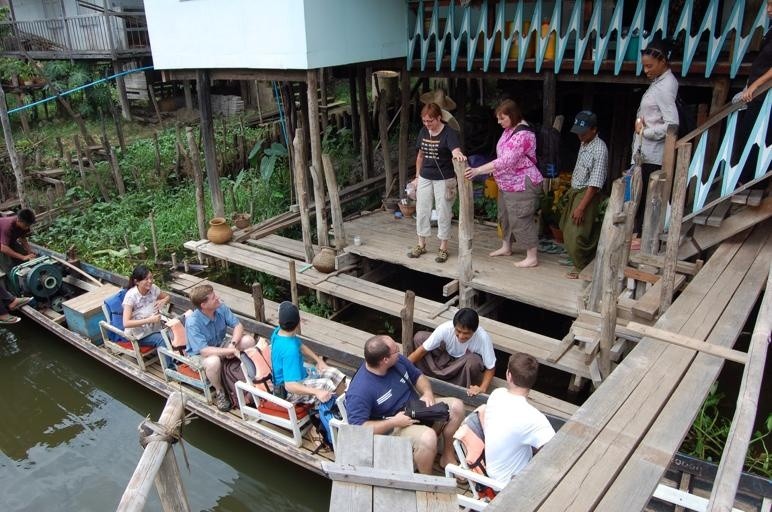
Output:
[
  {"left": 482, "top": 352, "right": 557, "bottom": 495},
  {"left": 270, "top": 301, "right": 346, "bottom": 453},
  {"left": 0, "top": 286, "right": 34, "bottom": 325},
  {"left": 464, "top": 98, "right": 545, "bottom": 268},
  {"left": 559, "top": 109, "right": 609, "bottom": 280},
  {"left": 0, "top": 209, "right": 36, "bottom": 277},
  {"left": 406, "top": 308, "right": 497, "bottom": 397},
  {"left": 344, "top": 334, "right": 468, "bottom": 484},
  {"left": 121, "top": 265, "right": 182, "bottom": 385},
  {"left": 630, "top": 39, "right": 680, "bottom": 251},
  {"left": 408, "top": 102, "right": 467, "bottom": 262},
  {"left": 184, "top": 285, "right": 256, "bottom": 411},
  {"left": 730, "top": 0, "right": 772, "bottom": 196}
]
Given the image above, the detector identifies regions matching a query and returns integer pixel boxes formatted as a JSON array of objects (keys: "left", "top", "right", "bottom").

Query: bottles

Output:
[
  {"left": 328, "top": 224, "right": 336, "bottom": 248},
  {"left": 206, "top": 218, "right": 234, "bottom": 243},
  {"left": 310, "top": 367, "right": 320, "bottom": 378},
  {"left": 312, "top": 245, "right": 337, "bottom": 274},
  {"left": 354, "top": 236, "right": 361, "bottom": 247}
]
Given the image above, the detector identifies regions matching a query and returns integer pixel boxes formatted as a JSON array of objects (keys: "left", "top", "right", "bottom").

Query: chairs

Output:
[
  {"left": 233, "top": 340, "right": 320, "bottom": 448},
  {"left": 444, "top": 403, "right": 504, "bottom": 512},
  {"left": 325, "top": 388, "right": 352, "bottom": 454},
  {"left": 97, "top": 283, "right": 171, "bottom": 370},
  {"left": 157, "top": 304, "right": 221, "bottom": 410}
]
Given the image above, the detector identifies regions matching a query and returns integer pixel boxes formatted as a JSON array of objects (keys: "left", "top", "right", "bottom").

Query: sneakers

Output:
[
  {"left": 407, "top": 245, "right": 426, "bottom": 258},
  {"left": 436, "top": 249, "right": 448, "bottom": 262},
  {"left": 216, "top": 389, "right": 231, "bottom": 412}
]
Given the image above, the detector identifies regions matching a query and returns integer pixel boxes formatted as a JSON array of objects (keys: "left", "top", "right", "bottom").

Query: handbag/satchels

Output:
[
  {"left": 140, "top": 300, "right": 170, "bottom": 331},
  {"left": 404, "top": 399, "right": 449, "bottom": 421},
  {"left": 444, "top": 178, "right": 457, "bottom": 200},
  {"left": 222, "top": 357, "right": 254, "bottom": 407},
  {"left": 319, "top": 395, "right": 343, "bottom": 444}
]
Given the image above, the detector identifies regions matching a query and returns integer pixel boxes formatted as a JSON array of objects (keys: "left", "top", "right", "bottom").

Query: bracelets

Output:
[{"left": 230, "top": 341, "right": 238, "bottom": 345}]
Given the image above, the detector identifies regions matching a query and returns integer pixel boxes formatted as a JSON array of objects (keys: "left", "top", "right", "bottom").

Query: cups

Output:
[{"left": 395, "top": 212, "right": 402, "bottom": 219}]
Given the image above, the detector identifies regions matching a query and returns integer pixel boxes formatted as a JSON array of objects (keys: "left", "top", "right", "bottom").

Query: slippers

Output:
[
  {"left": 0, "top": 314, "right": 21, "bottom": 324},
  {"left": 308, "top": 430, "right": 331, "bottom": 452},
  {"left": 10, "top": 297, "right": 34, "bottom": 311},
  {"left": 538, "top": 239, "right": 564, "bottom": 254}
]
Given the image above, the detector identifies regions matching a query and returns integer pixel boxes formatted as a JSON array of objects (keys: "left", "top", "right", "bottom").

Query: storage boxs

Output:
[{"left": 61, "top": 283, "right": 125, "bottom": 346}]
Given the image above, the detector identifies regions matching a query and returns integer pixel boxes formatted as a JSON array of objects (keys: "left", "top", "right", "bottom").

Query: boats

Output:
[{"left": 1, "top": 241, "right": 498, "bottom": 507}]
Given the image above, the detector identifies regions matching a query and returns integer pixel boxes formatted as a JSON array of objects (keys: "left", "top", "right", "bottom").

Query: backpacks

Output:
[{"left": 511, "top": 121, "right": 564, "bottom": 178}]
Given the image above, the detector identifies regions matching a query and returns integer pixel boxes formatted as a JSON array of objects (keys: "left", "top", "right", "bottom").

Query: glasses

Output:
[{"left": 387, "top": 345, "right": 400, "bottom": 355}]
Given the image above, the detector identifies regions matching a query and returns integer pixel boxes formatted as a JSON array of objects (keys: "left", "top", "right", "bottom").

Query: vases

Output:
[{"left": 206, "top": 216, "right": 235, "bottom": 245}]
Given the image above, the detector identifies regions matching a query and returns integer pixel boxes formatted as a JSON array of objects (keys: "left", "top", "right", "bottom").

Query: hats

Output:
[
  {"left": 571, "top": 110, "right": 597, "bottom": 134},
  {"left": 420, "top": 88, "right": 457, "bottom": 110},
  {"left": 441, "top": 109, "right": 460, "bottom": 132},
  {"left": 278, "top": 301, "right": 299, "bottom": 326},
  {"left": 406, "top": 177, "right": 418, "bottom": 200}
]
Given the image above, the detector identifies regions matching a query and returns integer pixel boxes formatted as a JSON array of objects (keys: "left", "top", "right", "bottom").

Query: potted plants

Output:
[{"left": 548, "top": 171, "right": 569, "bottom": 246}]
